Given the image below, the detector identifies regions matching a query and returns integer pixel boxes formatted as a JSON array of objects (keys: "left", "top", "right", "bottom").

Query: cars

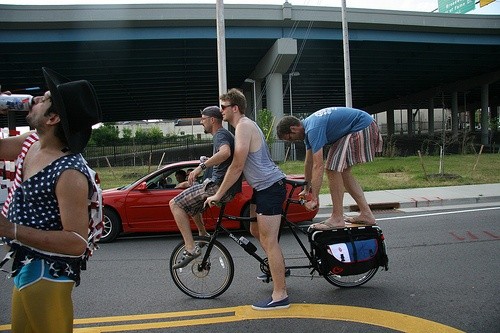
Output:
[{"left": 97, "top": 156, "right": 321, "bottom": 243}]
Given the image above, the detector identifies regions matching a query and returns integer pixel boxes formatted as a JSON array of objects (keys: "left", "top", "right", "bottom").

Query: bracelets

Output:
[{"left": 14, "top": 222, "right": 17, "bottom": 239}]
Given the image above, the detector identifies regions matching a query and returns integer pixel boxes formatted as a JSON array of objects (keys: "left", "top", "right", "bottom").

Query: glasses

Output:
[
  {"left": 222, "top": 105, "right": 235, "bottom": 111},
  {"left": 288, "top": 134, "right": 292, "bottom": 142},
  {"left": 43, "top": 91, "right": 52, "bottom": 102}
]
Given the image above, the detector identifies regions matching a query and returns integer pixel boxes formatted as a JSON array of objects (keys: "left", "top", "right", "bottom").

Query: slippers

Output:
[
  {"left": 314, "top": 221, "right": 345, "bottom": 230},
  {"left": 346, "top": 217, "right": 376, "bottom": 225}
]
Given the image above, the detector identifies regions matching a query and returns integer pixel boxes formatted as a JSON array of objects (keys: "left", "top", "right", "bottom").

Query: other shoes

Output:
[
  {"left": 257, "top": 268, "right": 288, "bottom": 280},
  {"left": 172, "top": 248, "right": 200, "bottom": 269},
  {"left": 196, "top": 233, "right": 210, "bottom": 247},
  {"left": 252, "top": 295, "right": 290, "bottom": 309}
]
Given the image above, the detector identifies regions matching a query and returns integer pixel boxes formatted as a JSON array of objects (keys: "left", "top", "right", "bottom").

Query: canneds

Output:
[
  {"left": 0, "top": 93, "right": 34, "bottom": 111},
  {"left": 200, "top": 156, "right": 208, "bottom": 163}
]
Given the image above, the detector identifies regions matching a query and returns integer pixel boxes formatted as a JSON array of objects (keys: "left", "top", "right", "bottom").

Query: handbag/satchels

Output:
[{"left": 315, "top": 227, "right": 389, "bottom": 276}]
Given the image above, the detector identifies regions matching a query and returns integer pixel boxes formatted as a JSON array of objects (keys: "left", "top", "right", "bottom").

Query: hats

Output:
[
  {"left": 42, "top": 68, "right": 103, "bottom": 154},
  {"left": 201, "top": 106, "right": 223, "bottom": 120}
]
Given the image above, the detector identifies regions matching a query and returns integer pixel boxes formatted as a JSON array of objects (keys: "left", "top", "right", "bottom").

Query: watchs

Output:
[{"left": 200, "top": 162, "right": 206, "bottom": 170}]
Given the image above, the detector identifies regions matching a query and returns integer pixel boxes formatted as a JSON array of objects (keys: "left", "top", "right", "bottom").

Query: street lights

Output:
[
  {"left": 289, "top": 72, "right": 300, "bottom": 149},
  {"left": 245, "top": 79, "right": 257, "bottom": 123}
]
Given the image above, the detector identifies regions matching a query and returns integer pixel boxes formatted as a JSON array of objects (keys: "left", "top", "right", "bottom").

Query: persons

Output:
[
  {"left": 203, "top": 88, "right": 291, "bottom": 309},
  {"left": 276, "top": 108, "right": 383, "bottom": 230},
  {"left": 169, "top": 106, "right": 242, "bottom": 269},
  {"left": 173, "top": 168, "right": 198, "bottom": 189},
  {"left": 0, "top": 68, "right": 100, "bottom": 333}
]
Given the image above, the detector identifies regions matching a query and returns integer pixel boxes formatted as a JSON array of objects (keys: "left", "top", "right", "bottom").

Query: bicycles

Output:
[{"left": 168, "top": 177, "right": 384, "bottom": 300}]
